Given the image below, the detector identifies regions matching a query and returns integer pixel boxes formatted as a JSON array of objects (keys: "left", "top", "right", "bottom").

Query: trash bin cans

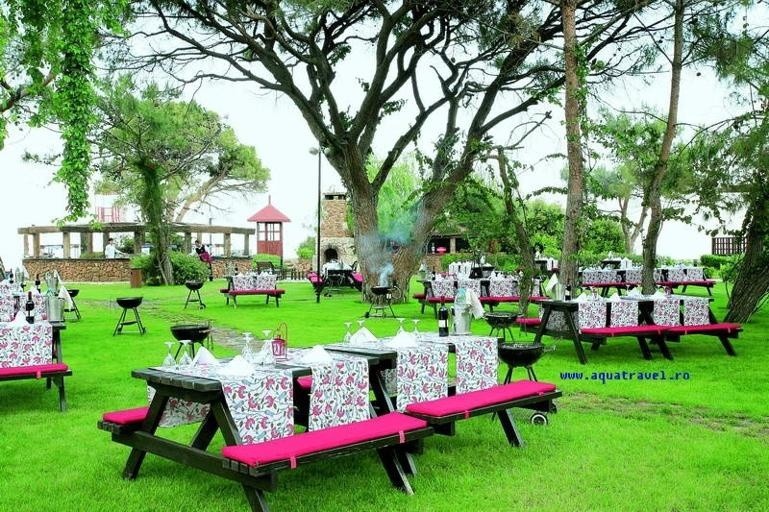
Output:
[
  {"left": 471, "top": 266, "right": 495, "bottom": 296},
  {"left": 600, "top": 260, "right": 621, "bottom": 269},
  {"left": 534, "top": 260, "right": 547, "bottom": 271}
]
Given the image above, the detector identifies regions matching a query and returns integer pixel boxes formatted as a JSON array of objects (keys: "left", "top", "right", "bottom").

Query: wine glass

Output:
[
  {"left": 238, "top": 328, "right": 275, "bottom": 368},
  {"left": 244, "top": 267, "right": 273, "bottom": 275},
  {"left": 177, "top": 338, "right": 195, "bottom": 371},
  {"left": 162, "top": 340, "right": 176, "bottom": 368},
  {"left": 357, "top": 319, "right": 364, "bottom": 329},
  {"left": 409, "top": 319, "right": 421, "bottom": 344},
  {"left": 396, "top": 318, "right": 405, "bottom": 335},
  {"left": 341, "top": 320, "right": 354, "bottom": 347}
]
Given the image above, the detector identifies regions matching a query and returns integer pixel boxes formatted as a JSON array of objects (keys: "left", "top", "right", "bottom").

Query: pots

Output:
[
  {"left": 186, "top": 280, "right": 204, "bottom": 290},
  {"left": 498, "top": 341, "right": 546, "bottom": 366},
  {"left": 116, "top": 296, "right": 143, "bottom": 310},
  {"left": 482, "top": 310, "right": 517, "bottom": 331},
  {"left": 370, "top": 286, "right": 391, "bottom": 297},
  {"left": 60, "top": 288, "right": 79, "bottom": 297},
  {"left": 170, "top": 324, "right": 212, "bottom": 343}
]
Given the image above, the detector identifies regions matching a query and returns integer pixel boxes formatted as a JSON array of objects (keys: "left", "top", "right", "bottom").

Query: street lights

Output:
[{"left": 307, "top": 145, "right": 326, "bottom": 303}]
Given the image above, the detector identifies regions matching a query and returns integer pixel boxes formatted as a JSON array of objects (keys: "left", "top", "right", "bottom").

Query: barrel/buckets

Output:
[
  {"left": 15, "top": 272, "right": 24, "bottom": 283},
  {"left": 454, "top": 303, "right": 473, "bottom": 334},
  {"left": 46, "top": 296, "right": 66, "bottom": 323},
  {"left": 554, "top": 284, "right": 565, "bottom": 301},
  {"left": 44, "top": 277, "right": 59, "bottom": 291}
]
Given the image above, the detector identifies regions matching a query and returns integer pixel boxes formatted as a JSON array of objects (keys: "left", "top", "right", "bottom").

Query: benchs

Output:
[
  {"left": 229, "top": 289, "right": 286, "bottom": 297},
  {"left": 219, "top": 287, "right": 229, "bottom": 294},
  {"left": 95, "top": 406, "right": 152, "bottom": 434},
  {"left": 415, "top": 251, "right": 744, "bottom": 365},
  {"left": 405, "top": 380, "right": 561, "bottom": 426},
  {"left": 219, "top": 411, "right": 433, "bottom": 479},
  {"left": 304, "top": 259, "right": 363, "bottom": 298},
  {"left": 298, "top": 377, "right": 315, "bottom": 390}
]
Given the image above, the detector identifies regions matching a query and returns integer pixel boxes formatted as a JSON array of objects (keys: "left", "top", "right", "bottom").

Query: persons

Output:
[
  {"left": 189, "top": 239, "right": 213, "bottom": 281},
  {"left": 105, "top": 239, "right": 125, "bottom": 259}
]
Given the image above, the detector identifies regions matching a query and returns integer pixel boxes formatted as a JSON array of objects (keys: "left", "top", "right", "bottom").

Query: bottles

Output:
[
  {"left": 438, "top": 295, "right": 449, "bottom": 337},
  {"left": 454, "top": 286, "right": 471, "bottom": 332},
  {"left": 7, "top": 267, "right": 41, "bottom": 325},
  {"left": 431, "top": 266, "right": 436, "bottom": 280},
  {"left": 564, "top": 276, "right": 572, "bottom": 301},
  {"left": 234, "top": 263, "right": 238, "bottom": 275}
]
